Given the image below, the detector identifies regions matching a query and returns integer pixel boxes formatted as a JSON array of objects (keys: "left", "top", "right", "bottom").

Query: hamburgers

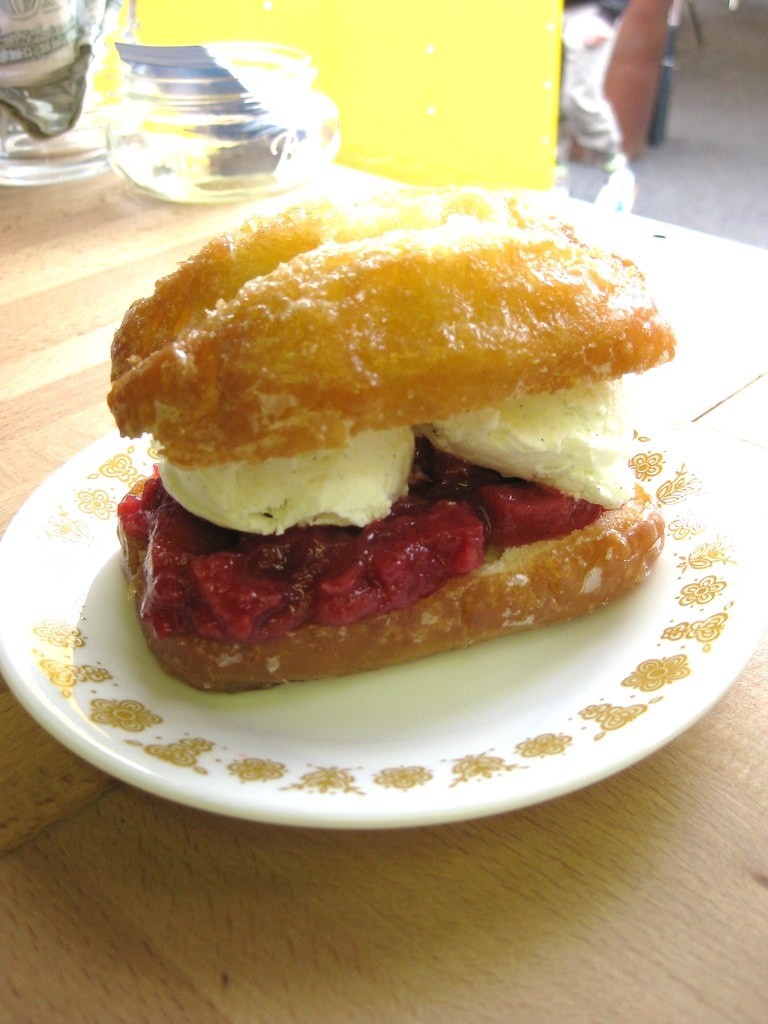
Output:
[{"left": 111, "top": 196, "right": 676, "bottom": 691}]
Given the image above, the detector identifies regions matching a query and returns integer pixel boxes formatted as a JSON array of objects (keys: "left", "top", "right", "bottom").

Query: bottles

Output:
[{"left": 106, "top": 38, "right": 341, "bottom": 207}]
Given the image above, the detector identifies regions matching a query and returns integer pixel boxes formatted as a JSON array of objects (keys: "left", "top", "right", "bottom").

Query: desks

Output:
[{"left": 1, "top": 172, "right": 767, "bottom": 1024}]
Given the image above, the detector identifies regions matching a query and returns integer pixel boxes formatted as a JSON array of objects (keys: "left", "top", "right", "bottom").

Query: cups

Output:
[{"left": 0, "top": 0, "right": 138, "bottom": 188}]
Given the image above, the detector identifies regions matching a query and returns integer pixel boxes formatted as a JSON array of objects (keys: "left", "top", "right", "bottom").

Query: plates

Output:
[{"left": 0, "top": 396, "right": 768, "bottom": 830}]
